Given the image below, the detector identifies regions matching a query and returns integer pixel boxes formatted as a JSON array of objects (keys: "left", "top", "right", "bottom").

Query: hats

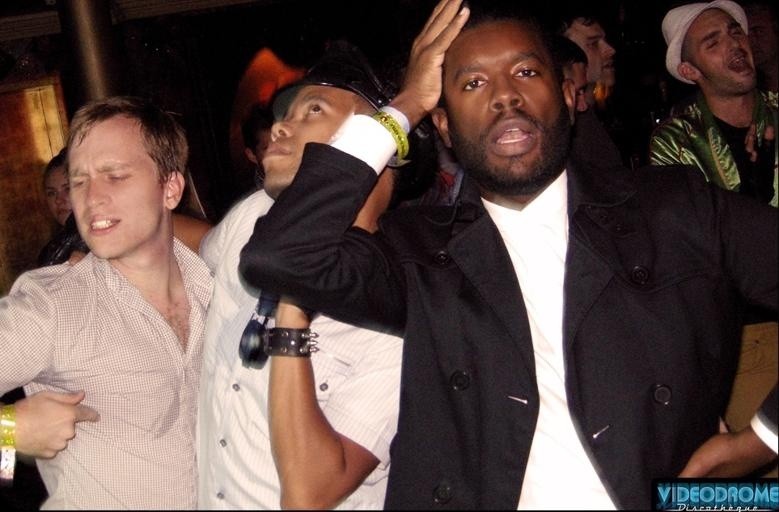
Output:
[
  {"left": 269, "top": 40, "right": 438, "bottom": 210},
  {"left": 662, "top": 0, "right": 749, "bottom": 85}
]
